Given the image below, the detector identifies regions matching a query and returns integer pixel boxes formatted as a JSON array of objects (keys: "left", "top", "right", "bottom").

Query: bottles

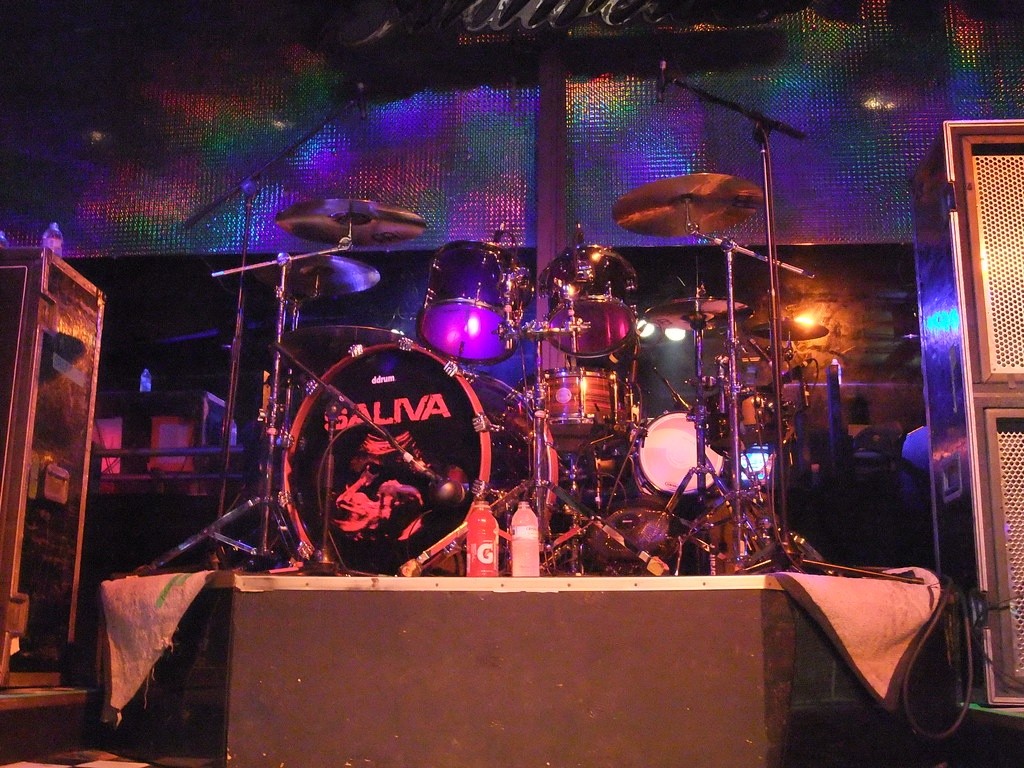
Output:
[
  {"left": 466, "top": 497, "right": 499, "bottom": 577},
  {"left": 511, "top": 501, "right": 540, "bottom": 576},
  {"left": 139, "top": 367, "right": 152, "bottom": 393}
]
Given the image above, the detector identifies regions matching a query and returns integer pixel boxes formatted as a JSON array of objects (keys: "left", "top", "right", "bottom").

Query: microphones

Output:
[
  {"left": 767, "top": 359, "right": 811, "bottom": 393},
  {"left": 657, "top": 58, "right": 669, "bottom": 104},
  {"left": 493, "top": 221, "right": 505, "bottom": 244},
  {"left": 574, "top": 222, "right": 584, "bottom": 245},
  {"left": 404, "top": 455, "right": 466, "bottom": 508}
]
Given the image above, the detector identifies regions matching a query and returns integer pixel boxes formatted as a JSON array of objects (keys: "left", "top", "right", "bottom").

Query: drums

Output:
[
  {"left": 414, "top": 239, "right": 524, "bottom": 367},
  {"left": 516, "top": 365, "right": 634, "bottom": 456},
  {"left": 630, "top": 409, "right": 726, "bottom": 501},
  {"left": 280, "top": 341, "right": 561, "bottom": 578},
  {"left": 538, "top": 242, "right": 640, "bottom": 359},
  {"left": 709, "top": 384, "right": 779, "bottom": 449}
]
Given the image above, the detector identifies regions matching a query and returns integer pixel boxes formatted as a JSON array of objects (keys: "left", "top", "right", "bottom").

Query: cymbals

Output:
[
  {"left": 752, "top": 315, "right": 830, "bottom": 342},
  {"left": 611, "top": 170, "right": 765, "bottom": 240},
  {"left": 644, "top": 278, "right": 756, "bottom": 333},
  {"left": 278, "top": 323, "right": 408, "bottom": 374},
  {"left": 274, "top": 197, "right": 429, "bottom": 248},
  {"left": 252, "top": 254, "right": 383, "bottom": 298}
]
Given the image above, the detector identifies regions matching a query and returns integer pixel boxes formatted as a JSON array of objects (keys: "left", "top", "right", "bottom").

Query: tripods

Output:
[{"left": 108, "top": 75, "right": 926, "bottom": 586}]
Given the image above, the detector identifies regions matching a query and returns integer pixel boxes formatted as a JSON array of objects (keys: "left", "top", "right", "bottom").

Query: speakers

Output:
[{"left": 905, "top": 119, "right": 1024, "bottom": 708}]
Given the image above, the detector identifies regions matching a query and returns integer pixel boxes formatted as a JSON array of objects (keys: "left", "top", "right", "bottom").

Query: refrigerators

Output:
[{"left": 0, "top": 245, "right": 107, "bottom": 689}]
